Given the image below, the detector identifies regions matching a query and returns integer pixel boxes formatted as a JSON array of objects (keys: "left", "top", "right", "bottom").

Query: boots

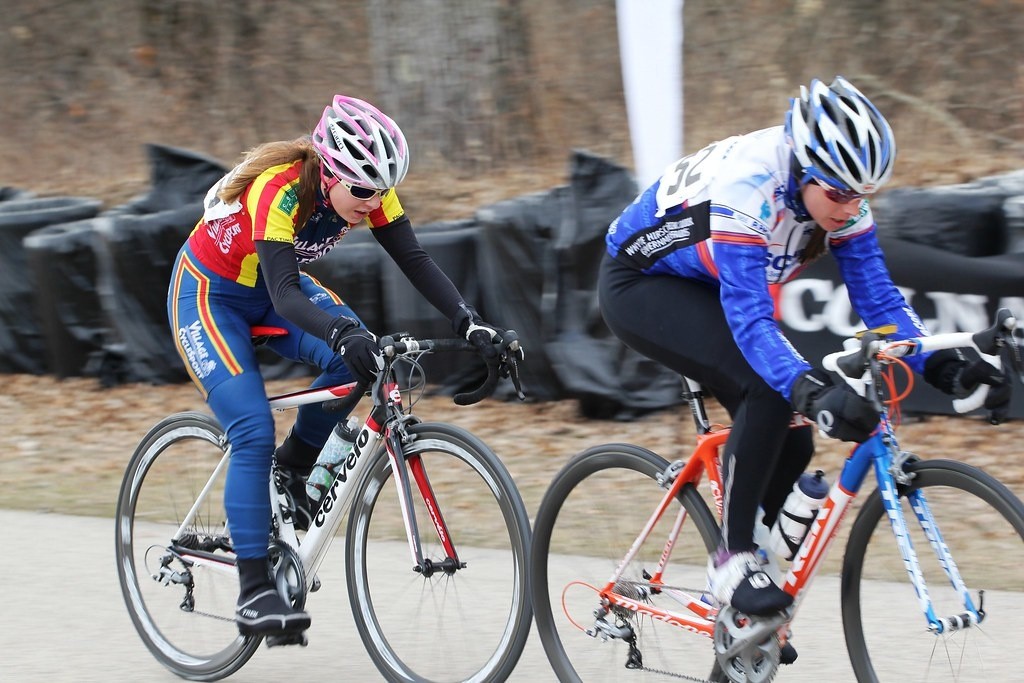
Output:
[
  {"left": 274, "top": 425, "right": 320, "bottom": 531},
  {"left": 234, "top": 556, "right": 311, "bottom": 635}
]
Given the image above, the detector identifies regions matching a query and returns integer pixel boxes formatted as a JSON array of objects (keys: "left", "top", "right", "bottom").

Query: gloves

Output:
[
  {"left": 327, "top": 314, "right": 386, "bottom": 388},
  {"left": 923, "top": 345, "right": 1015, "bottom": 423},
  {"left": 790, "top": 368, "right": 881, "bottom": 443},
  {"left": 452, "top": 301, "right": 525, "bottom": 380}
]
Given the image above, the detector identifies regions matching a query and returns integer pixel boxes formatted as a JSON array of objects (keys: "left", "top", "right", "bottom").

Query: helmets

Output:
[
  {"left": 312, "top": 95, "right": 411, "bottom": 190},
  {"left": 784, "top": 73, "right": 898, "bottom": 194}
]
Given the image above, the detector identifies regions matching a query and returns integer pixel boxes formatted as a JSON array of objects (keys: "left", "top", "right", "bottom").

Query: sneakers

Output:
[
  {"left": 777, "top": 643, "right": 798, "bottom": 665},
  {"left": 706, "top": 547, "right": 794, "bottom": 615}
]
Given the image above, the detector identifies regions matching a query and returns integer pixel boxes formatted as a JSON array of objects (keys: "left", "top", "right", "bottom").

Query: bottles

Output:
[
  {"left": 304, "top": 416, "right": 362, "bottom": 502},
  {"left": 767, "top": 469, "right": 828, "bottom": 557}
]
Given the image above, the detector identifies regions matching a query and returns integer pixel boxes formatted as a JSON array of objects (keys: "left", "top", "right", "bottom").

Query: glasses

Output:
[
  {"left": 318, "top": 152, "right": 389, "bottom": 201},
  {"left": 800, "top": 169, "right": 864, "bottom": 204}
]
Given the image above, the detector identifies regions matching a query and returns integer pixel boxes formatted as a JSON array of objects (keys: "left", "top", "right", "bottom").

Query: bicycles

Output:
[
  {"left": 527, "top": 304, "right": 1023, "bottom": 682},
  {"left": 110, "top": 318, "right": 546, "bottom": 683}
]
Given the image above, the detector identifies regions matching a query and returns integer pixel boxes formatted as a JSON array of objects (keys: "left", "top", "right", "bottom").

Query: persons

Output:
[
  {"left": 598, "top": 76, "right": 1012, "bottom": 665},
  {"left": 168, "top": 94, "right": 526, "bottom": 636}
]
